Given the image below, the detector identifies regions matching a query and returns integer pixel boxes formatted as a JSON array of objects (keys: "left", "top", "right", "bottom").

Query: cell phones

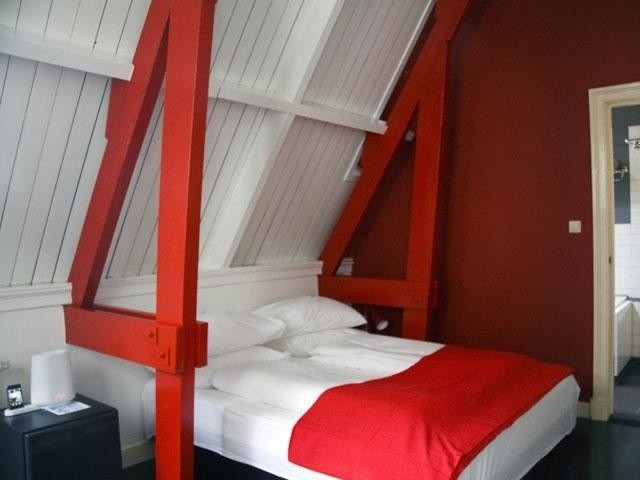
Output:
[{"left": 6, "top": 384, "right": 23, "bottom": 408}]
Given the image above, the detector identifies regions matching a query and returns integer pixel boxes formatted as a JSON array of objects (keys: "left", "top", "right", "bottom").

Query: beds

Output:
[{"left": 148, "top": 330, "right": 580, "bottom": 480}]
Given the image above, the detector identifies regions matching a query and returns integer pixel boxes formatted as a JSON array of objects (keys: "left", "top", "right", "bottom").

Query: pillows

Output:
[{"left": 195, "top": 292, "right": 369, "bottom": 390}]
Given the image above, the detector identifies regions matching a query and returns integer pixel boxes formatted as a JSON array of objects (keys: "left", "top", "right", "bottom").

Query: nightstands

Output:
[{"left": 0, "top": 394, "right": 124, "bottom": 478}]
